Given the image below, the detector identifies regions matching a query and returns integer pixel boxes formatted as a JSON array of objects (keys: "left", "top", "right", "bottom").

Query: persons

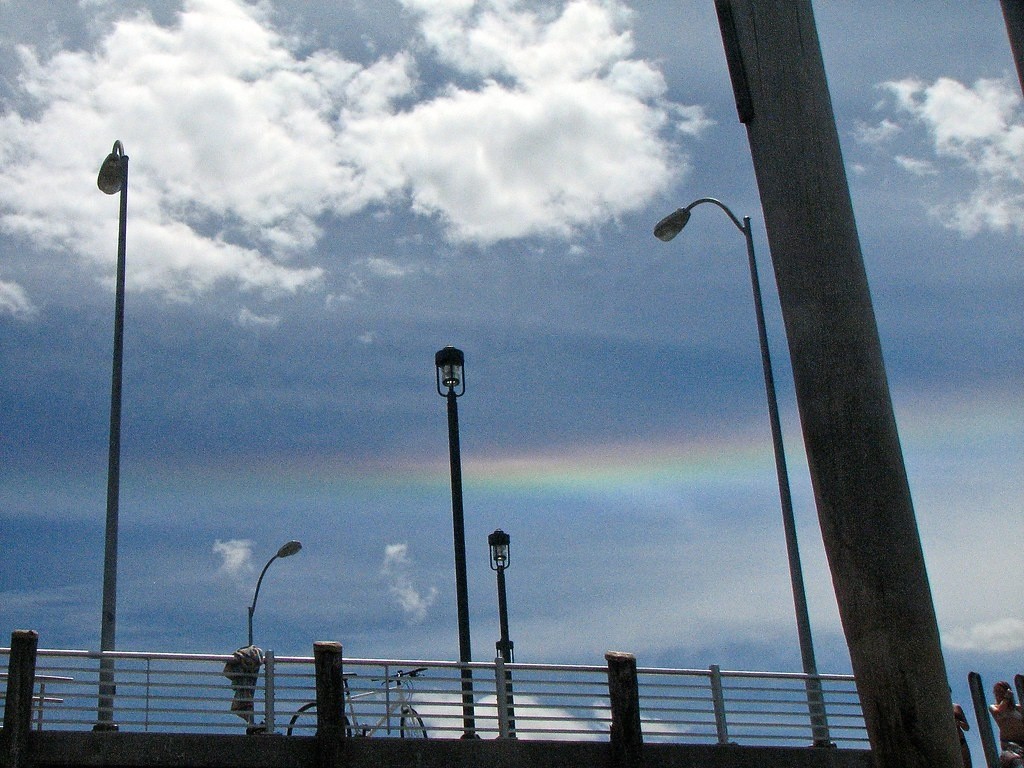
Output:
[
  {"left": 949, "top": 685, "right": 973, "bottom": 768},
  {"left": 988, "top": 680, "right": 1024, "bottom": 768}
]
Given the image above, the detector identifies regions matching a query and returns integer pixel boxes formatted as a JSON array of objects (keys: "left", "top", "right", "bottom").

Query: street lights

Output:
[
  {"left": 93, "top": 140, "right": 130, "bottom": 731},
  {"left": 654, "top": 197, "right": 837, "bottom": 748},
  {"left": 435, "top": 345, "right": 481, "bottom": 739},
  {"left": 488, "top": 529, "right": 515, "bottom": 736},
  {"left": 246, "top": 540, "right": 303, "bottom": 735}
]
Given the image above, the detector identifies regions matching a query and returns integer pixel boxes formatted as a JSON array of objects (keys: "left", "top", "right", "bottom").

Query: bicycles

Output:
[{"left": 287, "top": 667, "right": 428, "bottom": 738}]
